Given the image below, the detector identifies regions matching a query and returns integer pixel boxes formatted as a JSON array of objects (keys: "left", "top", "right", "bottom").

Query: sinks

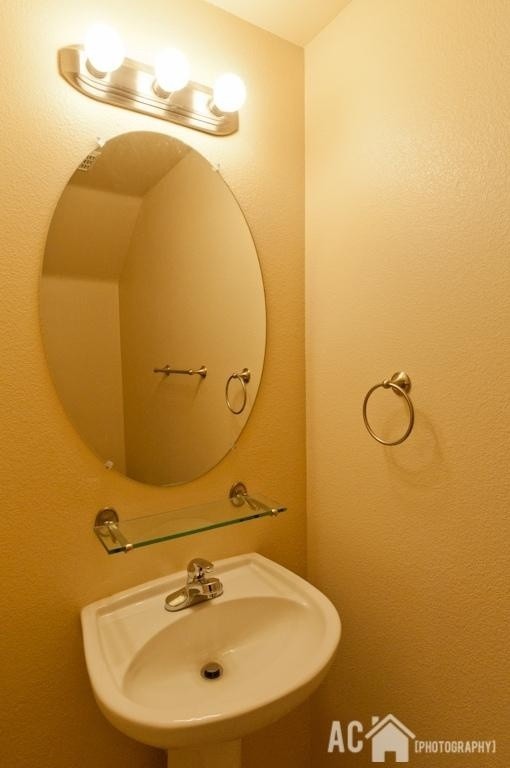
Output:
[{"left": 81, "top": 552, "right": 344, "bottom": 750}]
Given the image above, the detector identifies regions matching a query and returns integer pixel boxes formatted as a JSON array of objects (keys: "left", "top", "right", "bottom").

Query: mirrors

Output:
[{"left": 39, "top": 129, "right": 267, "bottom": 489}]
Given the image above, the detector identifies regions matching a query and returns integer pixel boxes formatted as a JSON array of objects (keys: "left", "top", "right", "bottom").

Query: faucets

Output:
[{"left": 187, "top": 557, "right": 223, "bottom": 605}]
